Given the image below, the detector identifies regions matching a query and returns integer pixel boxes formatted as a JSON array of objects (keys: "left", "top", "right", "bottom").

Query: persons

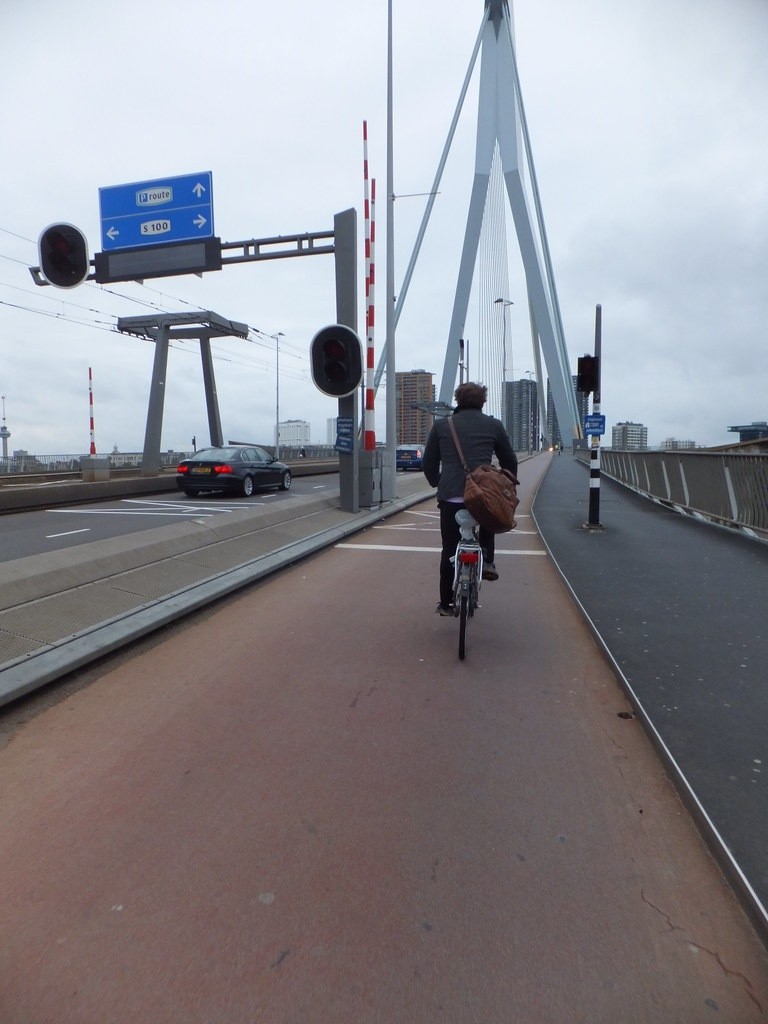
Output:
[{"left": 423, "top": 382, "right": 518, "bottom": 617}]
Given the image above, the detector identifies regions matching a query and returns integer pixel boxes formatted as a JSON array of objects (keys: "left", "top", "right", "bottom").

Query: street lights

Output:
[
  {"left": 524, "top": 369, "right": 535, "bottom": 382},
  {"left": 273, "top": 331, "right": 285, "bottom": 460},
  {"left": 495, "top": 298, "right": 514, "bottom": 382}
]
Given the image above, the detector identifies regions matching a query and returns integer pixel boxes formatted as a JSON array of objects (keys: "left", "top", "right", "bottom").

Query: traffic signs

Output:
[
  {"left": 99, "top": 171, "right": 215, "bottom": 252},
  {"left": 584, "top": 415, "right": 607, "bottom": 436},
  {"left": 333, "top": 415, "right": 358, "bottom": 454}
]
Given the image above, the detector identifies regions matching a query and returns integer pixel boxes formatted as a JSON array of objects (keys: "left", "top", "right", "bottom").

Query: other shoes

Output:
[
  {"left": 482, "top": 561, "right": 498, "bottom": 581},
  {"left": 433, "top": 601, "right": 455, "bottom": 616}
]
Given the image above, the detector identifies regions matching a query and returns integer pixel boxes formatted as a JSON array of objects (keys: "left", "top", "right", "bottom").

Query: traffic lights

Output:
[
  {"left": 458, "top": 337, "right": 466, "bottom": 351},
  {"left": 309, "top": 324, "right": 367, "bottom": 399},
  {"left": 191, "top": 436, "right": 196, "bottom": 445},
  {"left": 576, "top": 356, "right": 601, "bottom": 394},
  {"left": 37, "top": 222, "right": 92, "bottom": 291}
]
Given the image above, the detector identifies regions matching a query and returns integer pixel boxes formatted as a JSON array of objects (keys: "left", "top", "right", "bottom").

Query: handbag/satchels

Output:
[{"left": 466, "top": 464, "right": 521, "bottom": 535}]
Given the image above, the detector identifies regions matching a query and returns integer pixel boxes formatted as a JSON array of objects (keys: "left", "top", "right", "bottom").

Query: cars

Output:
[
  {"left": 397, "top": 441, "right": 426, "bottom": 473},
  {"left": 177, "top": 445, "right": 293, "bottom": 500}
]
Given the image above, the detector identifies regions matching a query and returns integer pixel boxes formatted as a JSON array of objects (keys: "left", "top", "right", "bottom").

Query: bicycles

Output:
[{"left": 448, "top": 507, "right": 485, "bottom": 658}]
